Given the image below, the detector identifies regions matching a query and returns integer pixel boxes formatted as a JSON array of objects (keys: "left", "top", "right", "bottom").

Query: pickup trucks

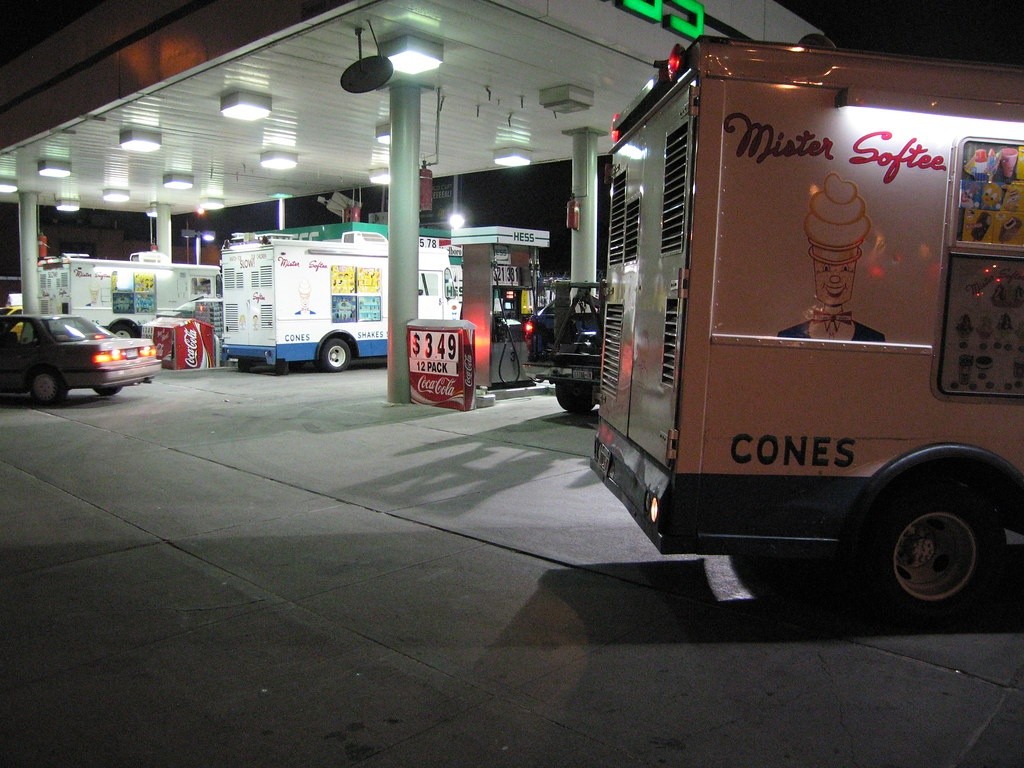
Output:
[{"left": 524, "top": 296, "right": 603, "bottom": 416}]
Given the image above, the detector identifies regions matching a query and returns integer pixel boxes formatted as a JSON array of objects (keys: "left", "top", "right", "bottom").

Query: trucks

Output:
[
  {"left": 220, "top": 230, "right": 462, "bottom": 373},
  {"left": 38, "top": 250, "right": 223, "bottom": 340},
  {"left": 589, "top": 34, "right": 1024, "bottom": 640}
]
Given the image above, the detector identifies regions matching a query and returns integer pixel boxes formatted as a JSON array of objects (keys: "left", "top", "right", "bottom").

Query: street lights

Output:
[{"left": 181, "top": 228, "right": 216, "bottom": 265}]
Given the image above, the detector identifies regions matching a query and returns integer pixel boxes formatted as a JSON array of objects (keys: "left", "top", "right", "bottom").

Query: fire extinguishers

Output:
[
  {"left": 566, "top": 192, "right": 580, "bottom": 230},
  {"left": 351, "top": 203, "right": 360, "bottom": 222},
  {"left": 150, "top": 238, "right": 158, "bottom": 252},
  {"left": 420, "top": 160, "right": 433, "bottom": 212},
  {"left": 344, "top": 204, "right": 352, "bottom": 222},
  {"left": 38, "top": 232, "right": 50, "bottom": 257}
]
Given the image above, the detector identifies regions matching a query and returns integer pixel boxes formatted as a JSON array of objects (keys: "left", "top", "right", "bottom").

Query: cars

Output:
[
  {"left": 0, "top": 304, "right": 24, "bottom": 341},
  {"left": 0, "top": 314, "right": 162, "bottom": 408}
]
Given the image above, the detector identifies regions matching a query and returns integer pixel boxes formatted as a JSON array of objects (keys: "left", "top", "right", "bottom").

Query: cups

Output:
[
  {"left": 957, "top": 354, "right": 993, "bottom": 390},
  {"left": 1000, "top": 147, "right": 1018, "bottom": 178}
]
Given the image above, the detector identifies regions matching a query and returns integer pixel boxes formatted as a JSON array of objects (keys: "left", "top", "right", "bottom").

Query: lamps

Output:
[
  {"left": 370, "top": 167, "right": 391, "bottom": 184},
  {"left": 201, "top": 197, "right": 226, "bottom": 210},
  {"left": 103, "top": 189, "right": 130, "bottom": 202},
  {"left": 119, "top": 129, "right": 162, "bottom": 151},
  {"left": 376, "top": 33, "right": 443, "bottom": 75},
  {"left": 37, "top": 160, "right": 72, "bottom": 177},
  {"left": 219, "top": 91, "right": 272, "bottom": 121},
  {"left": 0, "top": 180, "right": 17, "bottom": 192},
  {"left": 56, "top": 201, "right": 80, "bottom": 211},
  {"left": 266, "top": 186, "right": 295, "bottom": 199},
  {"left": 163, "top": 173, "right": 193, "bottom": 189},
  {"left": 261, "top": 149, "right": 297, "bottom": 170},
  {"left": 376, "top": 123, "right": 391, "bottom": 144},
  {"left": 494, "top": 149, "right": 532, "bottom": 166},
  {"left": 539, "top": 85, "right": 595, "bottom": 114}
]
